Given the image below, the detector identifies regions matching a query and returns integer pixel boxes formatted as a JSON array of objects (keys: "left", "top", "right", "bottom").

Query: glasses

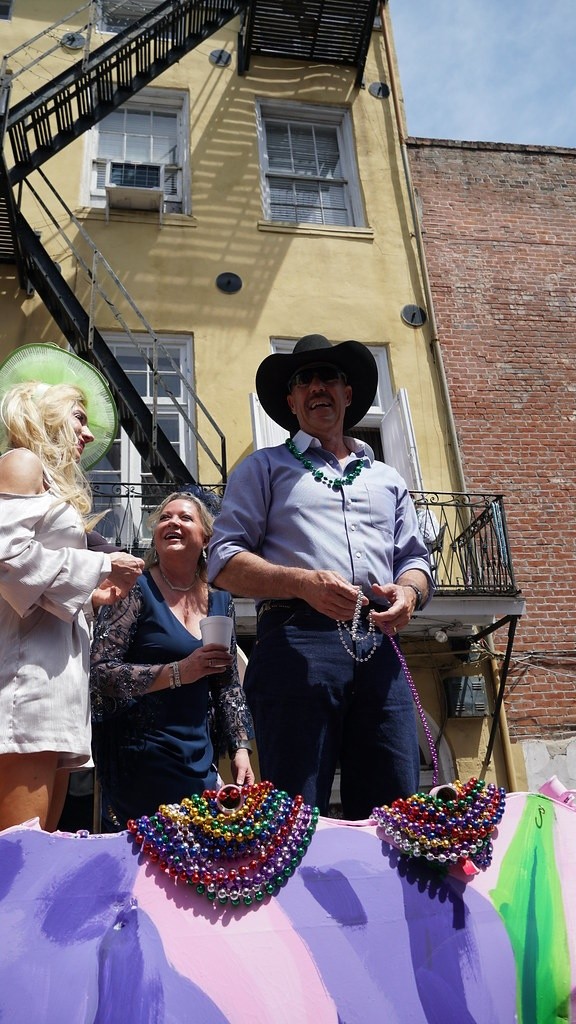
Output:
[{"left": 289, "top": 367, "right": 347, "bottom": 386}]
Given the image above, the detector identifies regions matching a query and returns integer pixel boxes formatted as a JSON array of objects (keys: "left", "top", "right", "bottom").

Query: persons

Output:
[
  {"left": 0, "top": 381, "right": 146, "bottom": 833},
  {"left": 408, "top": 493, "right": 439, "bottom": 582},
  {"left": 89, "top": 492, "right": 255, "bottom": 831},
  {"left": 201, "top": 336, "right": 435, "bottom": 818}
]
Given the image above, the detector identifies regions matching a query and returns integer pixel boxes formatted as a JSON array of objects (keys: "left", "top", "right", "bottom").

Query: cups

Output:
[{"left": 199, "top": 615, "right": 234, "bottom": 654}]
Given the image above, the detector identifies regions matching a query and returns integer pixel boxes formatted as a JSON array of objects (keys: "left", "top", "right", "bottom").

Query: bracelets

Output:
[
  {"left": 406, "top": 584, "right": 422, "bottom": 611},
  {"left": 168, "top": 661, "right": 182, "bottom": 689}
]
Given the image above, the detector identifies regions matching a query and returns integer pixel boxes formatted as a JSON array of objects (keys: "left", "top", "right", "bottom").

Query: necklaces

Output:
[
  {"left": 127, "top": 779, "right": 318, "bottom": 906},
  {"left": 381, "top": 622, "right": 439, "bottom": 788},
  {"left": 367, "top": 775, "right": 507, "bottom": 867},
  {"left": 160, "top": 571, "right": 199, "bottom": 592},
  {"left": 335, "top": 588, "right": 377, "bottom": 662},
  {"left": 283, "top": 434, "right": 365, "bottom": 487}
]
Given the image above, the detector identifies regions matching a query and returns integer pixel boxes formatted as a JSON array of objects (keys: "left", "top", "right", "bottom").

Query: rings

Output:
[
  {"left": 212, "top": 659, "right": 216, "bottom": 666},
  {"left": 393, "top": 627, "right": 397, "bottom": 633},
  {"left": 208, "top": 660, "right": 212, "bottom": 666}
]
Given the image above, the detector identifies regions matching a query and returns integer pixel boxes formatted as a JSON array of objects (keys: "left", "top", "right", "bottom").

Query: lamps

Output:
[{"left": 435, "top": 628, "right": 451, "bottom": 644}]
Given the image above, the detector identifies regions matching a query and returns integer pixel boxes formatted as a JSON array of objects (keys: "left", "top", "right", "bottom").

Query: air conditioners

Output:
[{"left": 104, "top": 159, "right": 165, "bottom": 212}]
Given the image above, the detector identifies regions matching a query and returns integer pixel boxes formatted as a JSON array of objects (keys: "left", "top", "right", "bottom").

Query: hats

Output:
[
  {"left": 255, "top": 334, "right": 378, "bottom": 434},
  {"left": 0, "top": 342, "right": 118, "bottom": 477}
]
